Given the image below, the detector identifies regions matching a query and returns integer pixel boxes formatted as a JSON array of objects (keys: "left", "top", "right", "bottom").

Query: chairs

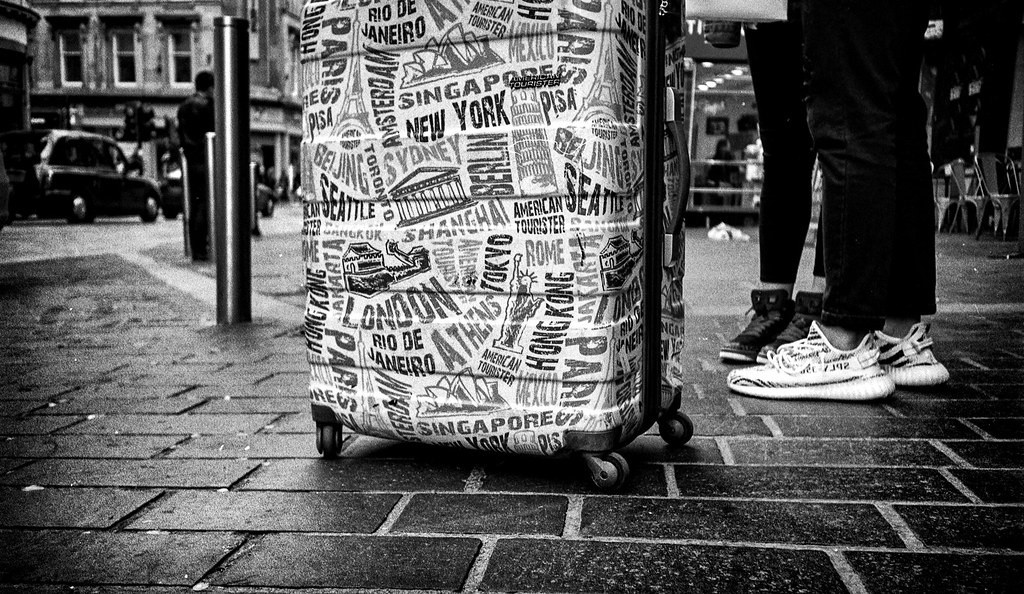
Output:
[{"left": 936, "top": 152, "right": 1019, "bottom": 245}]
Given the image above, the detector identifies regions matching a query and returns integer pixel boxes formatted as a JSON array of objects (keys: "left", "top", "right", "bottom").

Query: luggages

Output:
[{"left": 297, "top": 0, "right": 696, "bottom": 493}]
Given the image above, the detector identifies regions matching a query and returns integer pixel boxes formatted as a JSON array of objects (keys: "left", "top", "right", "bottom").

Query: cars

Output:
[
  {"left": 160, "top": 168, "right": 274, "bottom": 219},
  {"left": 0, "top": 129, "right": 162, "bottom": 225}
]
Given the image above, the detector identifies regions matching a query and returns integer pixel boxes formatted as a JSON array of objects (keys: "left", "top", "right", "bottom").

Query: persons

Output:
[
  {"left": 177, "top": 70, "right": 215, "bottom": 263},
  {"left": 703, "top": 0, "right": 951, "bottom": 403}
]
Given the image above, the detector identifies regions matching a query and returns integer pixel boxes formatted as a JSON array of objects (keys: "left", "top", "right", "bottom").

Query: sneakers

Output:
[
  {"left": 728, "top": 320, "right": 897, "bottom": 401},
  {"left": 718, "top": 288, "right": 795, "bottom": 364},
  {"left": 873, "top": 320, "right": 951, "bottom": 387},
  {"left": 756, "top": 289, "right": 827, "bottom": 366}
]
